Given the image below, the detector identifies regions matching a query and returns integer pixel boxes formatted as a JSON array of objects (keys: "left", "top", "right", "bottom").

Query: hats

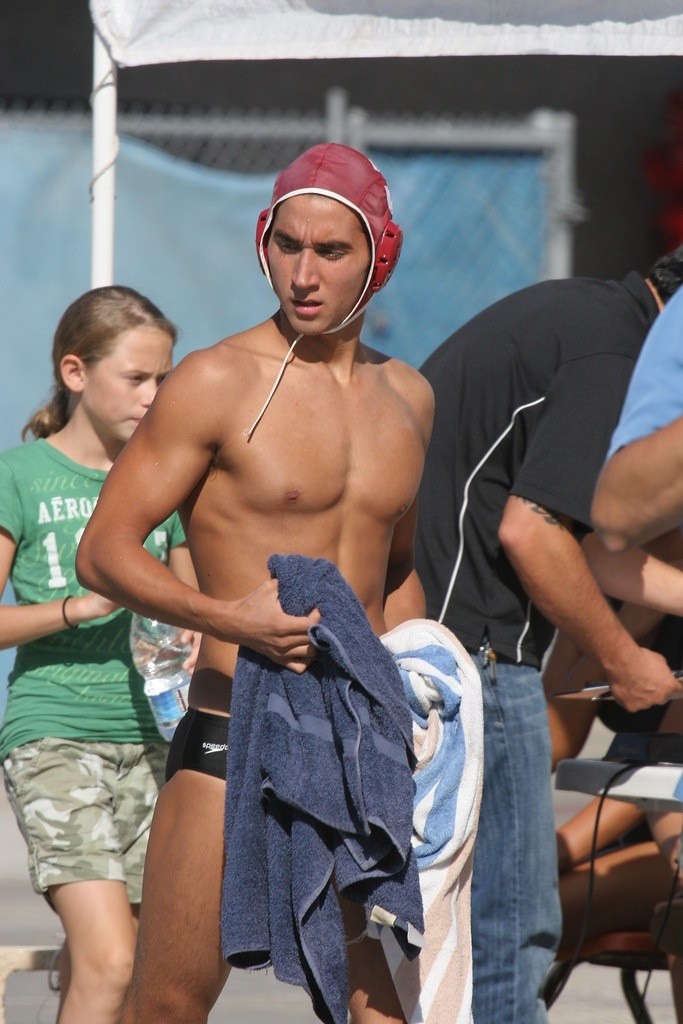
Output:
[{"left": 254, "top": 138, "right": 404, "bottom": 334}]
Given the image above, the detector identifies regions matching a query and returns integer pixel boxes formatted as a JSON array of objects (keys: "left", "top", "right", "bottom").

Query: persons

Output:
[
  {"left": 75, "top": 145, "right": 436, "bottom": 1024},
  {"left": 0, "top": 285, "right": 204, "bottom": 1024},
  {"left": 415, "top": 249, "right": 683, "bottom": 1024}
]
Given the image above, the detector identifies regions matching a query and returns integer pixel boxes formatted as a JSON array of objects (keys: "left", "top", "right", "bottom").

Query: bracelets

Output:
[{"left": 62, "top": 595, "right": 79, "bottom": 630}]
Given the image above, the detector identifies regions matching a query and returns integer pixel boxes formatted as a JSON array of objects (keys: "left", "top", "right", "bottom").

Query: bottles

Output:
[{"left": 129, "top": 611, "right": 193, "bottom": 742}]
